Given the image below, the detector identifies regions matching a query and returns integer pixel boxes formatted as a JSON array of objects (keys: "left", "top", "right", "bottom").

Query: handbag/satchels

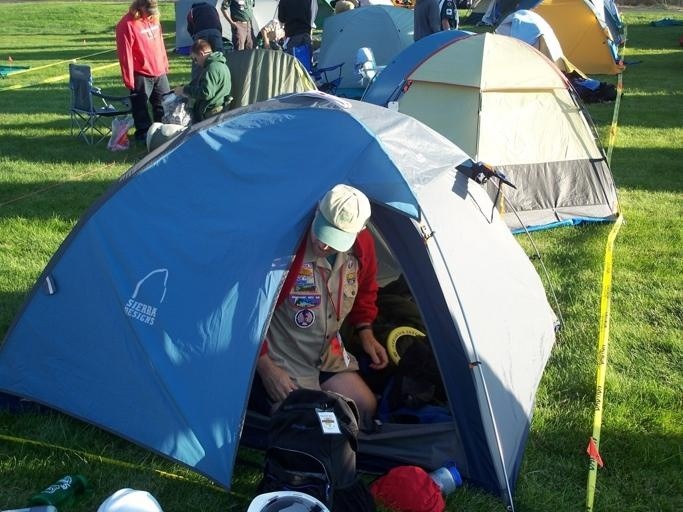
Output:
[{"left": 565, "top": 69, "right": 616, "bottom": 101}]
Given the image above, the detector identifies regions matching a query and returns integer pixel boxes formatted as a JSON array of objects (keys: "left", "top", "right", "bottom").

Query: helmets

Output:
[
  {"left": 96, "top": 487, "right": 163, "bottom": 512},
  {"left": 247, "top": 491, "right": 328, "bottom": 511}
]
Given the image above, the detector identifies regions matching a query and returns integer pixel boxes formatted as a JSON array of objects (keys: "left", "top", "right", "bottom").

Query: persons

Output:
[
  {"left": 172, "top": 38, "right": 232, "bottom": 126},
  {"left": 257, "top": 183, "right": 388, "bottom": 422},
  {"left": 278, "top": 1, "right": 312, "bottom": 51},
  {"left": 414, "top": 0, "right": 466, "bottom": 42},
  {"left": 187, "top": 3, "right": 222, "bottom": 55},
  {"left": 334, "top": 2, "right": 355, "bottom": 14},
  {"left": 257, "top": 20, "right": 284, "bottom": 50},
  {"left": 117, "top": 1, "right": 169, "bottom": 142},
  {"left": 220, "top": 1, "right": 255, "bottom": 51}
]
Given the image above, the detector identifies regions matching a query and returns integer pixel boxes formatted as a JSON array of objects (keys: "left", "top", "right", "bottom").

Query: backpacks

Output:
[{"left": 258, "top": 390, "right": 376, "bottom": 512}]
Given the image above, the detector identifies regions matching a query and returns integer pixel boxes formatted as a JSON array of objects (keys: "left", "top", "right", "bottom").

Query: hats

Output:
[
  {"left": 311, "top": 183, "right": 371, "bottom": 252},
  {"left": 139, "top": 0, "right": 159, "bottom": 15}
]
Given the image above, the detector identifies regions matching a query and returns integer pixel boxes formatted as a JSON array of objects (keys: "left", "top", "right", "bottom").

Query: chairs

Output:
[
  {"left": 287, "top": 30, "right": 345, "bottom": 96},
  {"left": 68, "top": 63, "right": 133, "bottom": 147}
]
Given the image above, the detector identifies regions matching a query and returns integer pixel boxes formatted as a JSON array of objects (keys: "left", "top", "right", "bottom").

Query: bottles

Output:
[
  {"left": 28, "top": 475, "right": 88, "bottom": 508},
  {"left": 429, "top": 467, "right": 463, "bottom": 495}
]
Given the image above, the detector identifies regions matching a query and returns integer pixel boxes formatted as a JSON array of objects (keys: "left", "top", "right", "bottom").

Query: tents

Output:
[
  {"left": 363, "top": 28, "right": 476, "bottom": 105},
  {"left": 0, "top": 93, "right": 562, "bottom": 505},
  {"left": 496, "top": 10, "right": 593, "bottom": 93},
  {"left": 224, "top": 49, "right": 318, "bottom": 108},
  {"left": 175, "top": 1, "right": 288, "bottom": 50},
  {"left": 520, "top": 0, "right": 625, "bottom": 76},
  {"left": 319, "top": 5, "right": 420, "bottom": 95},
  {"left": 387, "top": 32, "right": 620, "bottom": 232}
]
